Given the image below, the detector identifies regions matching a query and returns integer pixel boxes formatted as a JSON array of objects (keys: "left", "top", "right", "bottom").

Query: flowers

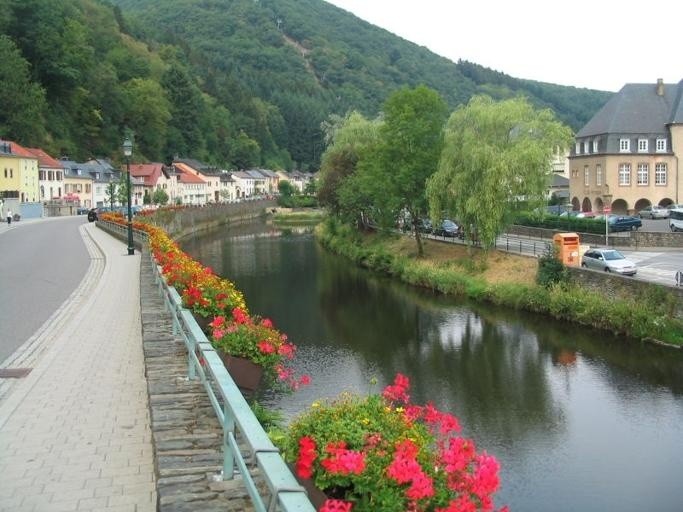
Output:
[
  {"left": 97, "top": 211, "right": 249, "bottom": 318},
  {"left": 271, "top": 377, "right": 512, "bottom": 512},
  {"left": 204, "top": 310, "right": 311, "bottom": 396}
]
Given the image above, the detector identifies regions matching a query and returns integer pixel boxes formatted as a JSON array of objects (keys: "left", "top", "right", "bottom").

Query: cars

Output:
[
  {"left": 396, "top": 209, "right": 480, "bottom": 242},
  {"left": 529, "top": 204, "right": 641, "bottom": 233},
  {"left": 637, "top": 204, "right": 682, "bottom": 232},
  {"left": 78, "top": 207, "right": 89, "bottom": 215},
  {"left": 581, "top": 248, "right": 637, "bottom": 276},
  {"left": 86, "top": 206, "right": 142, "bottom": 222}
]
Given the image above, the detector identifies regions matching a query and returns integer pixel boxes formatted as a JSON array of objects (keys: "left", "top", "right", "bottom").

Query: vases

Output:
[
  {"left": 219, "top": 351, "right": 265, "bottom": 391},
  {"left": 289, "top": 459, "right": 329, "bottom": 511},
  {"left": 192, "top": 310, "right": 215, "bottom": 333}
]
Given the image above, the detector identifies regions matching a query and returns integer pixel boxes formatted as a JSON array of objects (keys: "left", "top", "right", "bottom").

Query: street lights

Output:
[{"left": 121, "top": 136, "right": 138, "bottom": 255}]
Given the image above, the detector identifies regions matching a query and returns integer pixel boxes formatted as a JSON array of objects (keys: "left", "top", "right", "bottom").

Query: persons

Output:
[{"left": 6, "top": 207, "right": 13, "bottom": 224}]
